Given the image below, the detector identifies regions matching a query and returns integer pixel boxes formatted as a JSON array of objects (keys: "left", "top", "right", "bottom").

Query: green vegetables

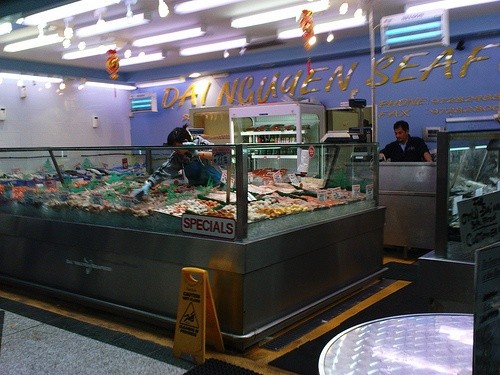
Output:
[{"left": 0, "top": 159, "right": 235, "bottom": 211}]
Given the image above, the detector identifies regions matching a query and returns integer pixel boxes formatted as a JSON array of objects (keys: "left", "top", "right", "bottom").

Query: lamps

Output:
[
  {"left": 0, "top": 70, "right": 63, "bottom": 84},
  {"left": 85, "top": 82, "right": 137, "bottom": 91},
  {"left": 403, "top": 0, "right": 498, "bottom": 13},
  {"left": 0, "top": 0, "right": 367, "bottom": 67},
  {"left": 135, "top": 75, "right": 185, "bottom": 88},
  {"left": 380, "top": 7, "right": 449, "bottom": 53},
  {"left": 127, "top": 94, "right": 157, "bottom": 113}
]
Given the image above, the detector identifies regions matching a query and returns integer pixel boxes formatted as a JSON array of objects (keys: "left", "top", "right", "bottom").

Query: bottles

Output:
[{"left": 249, "top": 135, "right": 296, "bottom": 143}]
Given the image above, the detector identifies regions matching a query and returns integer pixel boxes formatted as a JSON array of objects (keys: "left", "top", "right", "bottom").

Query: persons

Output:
[
  {"left": 381, "top": 120, "right": 436, "bottom": 162},
  {"left": 130, "top": 127, "right": 222, "bottom": 202}
]
